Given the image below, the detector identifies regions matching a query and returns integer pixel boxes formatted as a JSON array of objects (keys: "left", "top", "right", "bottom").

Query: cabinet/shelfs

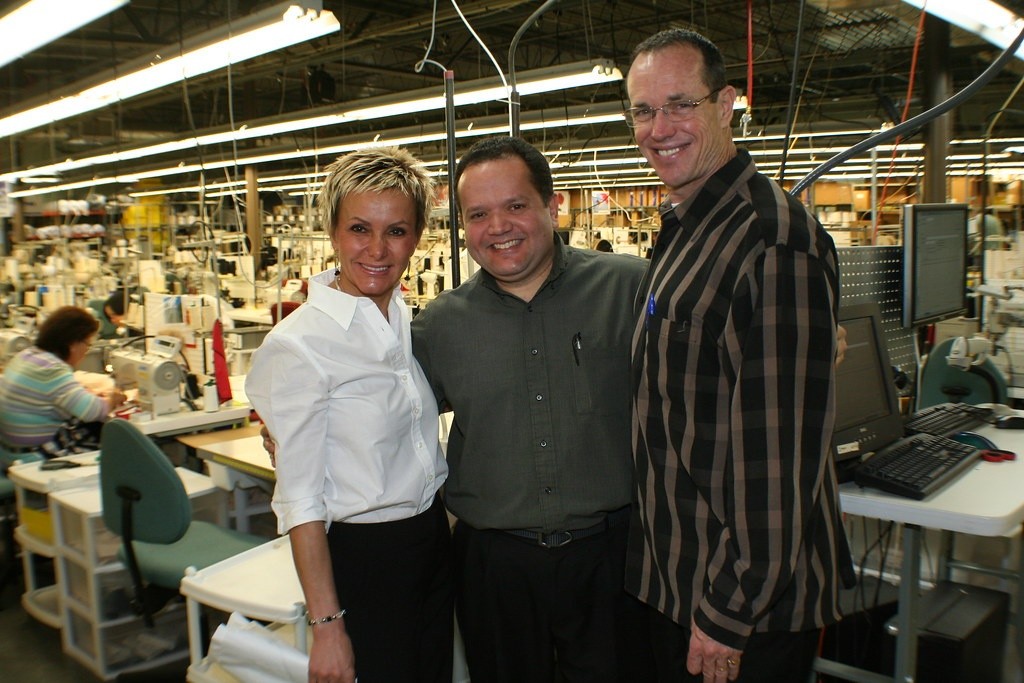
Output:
[
  {"left": 179, "top": 534, "right": 315, "bottom": 683},
  {"left": 8, "top": 449, "right": 101, "bottom": 628},
  {"left": 48, "top": 466, "right": 228, "bottom": 681}
]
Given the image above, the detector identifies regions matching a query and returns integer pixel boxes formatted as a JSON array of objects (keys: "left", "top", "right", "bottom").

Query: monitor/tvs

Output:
[
  {"left": 832, "top": 302, "right": 905, "bottom": 483},
  {"left": 901, "top": 203, "right": 968, "bottom": 329}
]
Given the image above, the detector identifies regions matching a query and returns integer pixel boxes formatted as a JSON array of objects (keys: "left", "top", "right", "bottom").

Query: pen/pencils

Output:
[
  {"left": 644, "top": 293, "right": 655, "bottom": 331},
  {"left": 571, "top": 332, "right": 581, "bottom": 367}
]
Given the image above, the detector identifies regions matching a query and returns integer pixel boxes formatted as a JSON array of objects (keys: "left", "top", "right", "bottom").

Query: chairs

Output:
[{"left": 99, "top": 419, "right": 275, "bottom": 658}]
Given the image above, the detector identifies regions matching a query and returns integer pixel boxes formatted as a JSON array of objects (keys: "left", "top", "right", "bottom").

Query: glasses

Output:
[{"left": 622, "top": 88, "right": 723, "bottom": 128}]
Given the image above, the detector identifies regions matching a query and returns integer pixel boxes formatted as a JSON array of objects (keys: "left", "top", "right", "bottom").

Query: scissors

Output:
[{"left": 981, "top": 448, "right": 1016, "bottom": 463}]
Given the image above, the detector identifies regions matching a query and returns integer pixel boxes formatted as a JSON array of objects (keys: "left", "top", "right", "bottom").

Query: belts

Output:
[{"left": 502, "top": 507, "right": 632, "bottom": 547}]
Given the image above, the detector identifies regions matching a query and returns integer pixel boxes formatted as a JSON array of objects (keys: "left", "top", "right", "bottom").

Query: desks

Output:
[
  {"left": 175, "top": 424, "right": 274, "bottom": 535},
  {"left": 813, "top": 409, "right": 1023, "bottom": 683}
]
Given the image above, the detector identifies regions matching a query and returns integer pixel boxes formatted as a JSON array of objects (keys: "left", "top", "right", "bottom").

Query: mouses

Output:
[
  {"left": 996, "top": 415, "right": 1024, "bottom": 430},
  {"left": 948, "top": 431, "right": 998, "bottom": 451}
]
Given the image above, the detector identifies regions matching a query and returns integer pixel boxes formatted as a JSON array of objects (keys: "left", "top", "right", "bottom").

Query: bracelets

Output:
[{"left": 307, "top": 608, "right": 347, "bottom": 626}]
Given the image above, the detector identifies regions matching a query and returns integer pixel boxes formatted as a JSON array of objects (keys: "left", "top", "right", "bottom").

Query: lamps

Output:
[{"left": 0, "top": 0, "right": 1024, "bottom": 197}]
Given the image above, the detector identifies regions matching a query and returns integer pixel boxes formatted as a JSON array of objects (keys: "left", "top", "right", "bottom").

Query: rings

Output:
[
  {"left": 717, "top": 666, "right": 729, "bottom": 671},
  {"left": 727, "top": 659, "right": 739, "bottom": 665}
]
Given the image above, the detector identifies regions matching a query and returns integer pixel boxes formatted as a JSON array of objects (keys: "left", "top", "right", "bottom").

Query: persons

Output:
[
  {"left": 96, "top": 291, "right": 129, "bottom": 340},
  {"left": 966, "top": 195, "right": 1006, "bottom": 255},
  {"left": 0, "top": 307, "right": 127, "bottom": 475},
  {"left": 245, "top": 126, "right": 455, "bottom": 682},
  {"left": 260, "top": 130, "right": 847, "bottom": 683},
  {"left": 622, "top": 31, "right": 862, "bottom": 682},
  {"left": 596, "top": 239, "right": 614, "bottom": 252}
]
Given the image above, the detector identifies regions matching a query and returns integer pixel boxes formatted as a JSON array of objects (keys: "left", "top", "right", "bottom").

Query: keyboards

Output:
[
  {"left": 902, "top": 401, "right": 996, "bottom": 438},
  {"left": 855, "top": 433, "right": 982, "bottom": 500}
]
